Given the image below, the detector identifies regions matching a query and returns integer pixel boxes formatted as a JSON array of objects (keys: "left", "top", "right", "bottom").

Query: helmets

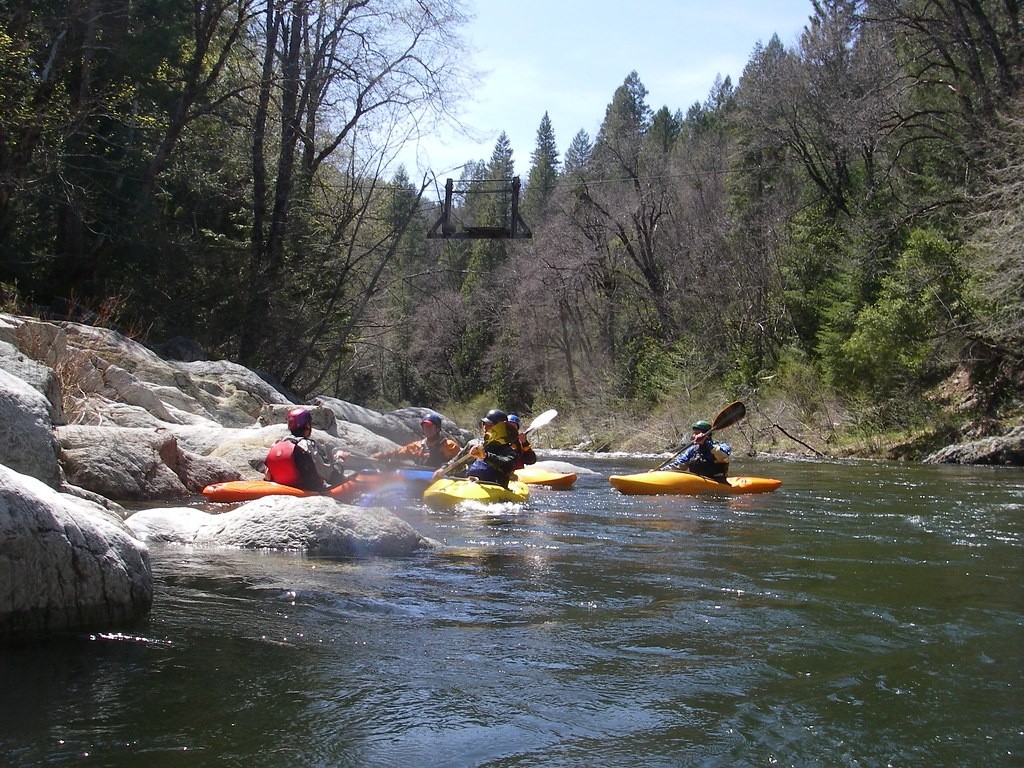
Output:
[
  {"left": 507, "top": 414, "right": 520, "bottom": 430},
  {"left": 287, "top": 409, "right": 312, "bottom": 431},
  {"left": 692, "top": 421, "right": 712, "bottom": 436},
  {"left": 421, "top": 414, "right": 441, "bottom": 431},
  {"left": 480, "top": 409, "right": 508, "bottom": 426}
]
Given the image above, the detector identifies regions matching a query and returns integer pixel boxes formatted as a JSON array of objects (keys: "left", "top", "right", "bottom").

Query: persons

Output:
[
  {"left": 506, "top": 414, "right": 537, "bottom": 469},
  {"left": 371, "top": 414, "right": 461, "bottom": 467},
  {"left": 649, "top": 420, "right": 732, "bottom": 485},
  {"left": 432, "top": 410, "right": 518, "bottom": 482},
  {"left": 265, "top": 409, "right": 349, "bottom": 490}
]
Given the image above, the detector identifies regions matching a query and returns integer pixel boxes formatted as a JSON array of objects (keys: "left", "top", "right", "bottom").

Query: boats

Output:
[
  {"left": 201, "top": 473, "right": 404, "bottom": 502},
  {"left": 358, "top": 467, "right": 448, "bottom": 505},
  {"left": 515, "top": 468, "right": 577, "bottom": 487},
  {"left": 422, "top": 478, "right": 531, "bottom": 510},
  {"left": 608, "top": 470, "right": 785, "bottom": 495}
]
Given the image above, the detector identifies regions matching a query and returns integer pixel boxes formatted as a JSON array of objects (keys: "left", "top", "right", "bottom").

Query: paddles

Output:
[
  {"left": 432, "top": 420, "right": 519, "bottom": 481},
  {"left": 654, "top": 401, "right": 747, "bottom": 474},
  {"left": 505, "top": 408, "right": 559, "bottom": 448}
]
